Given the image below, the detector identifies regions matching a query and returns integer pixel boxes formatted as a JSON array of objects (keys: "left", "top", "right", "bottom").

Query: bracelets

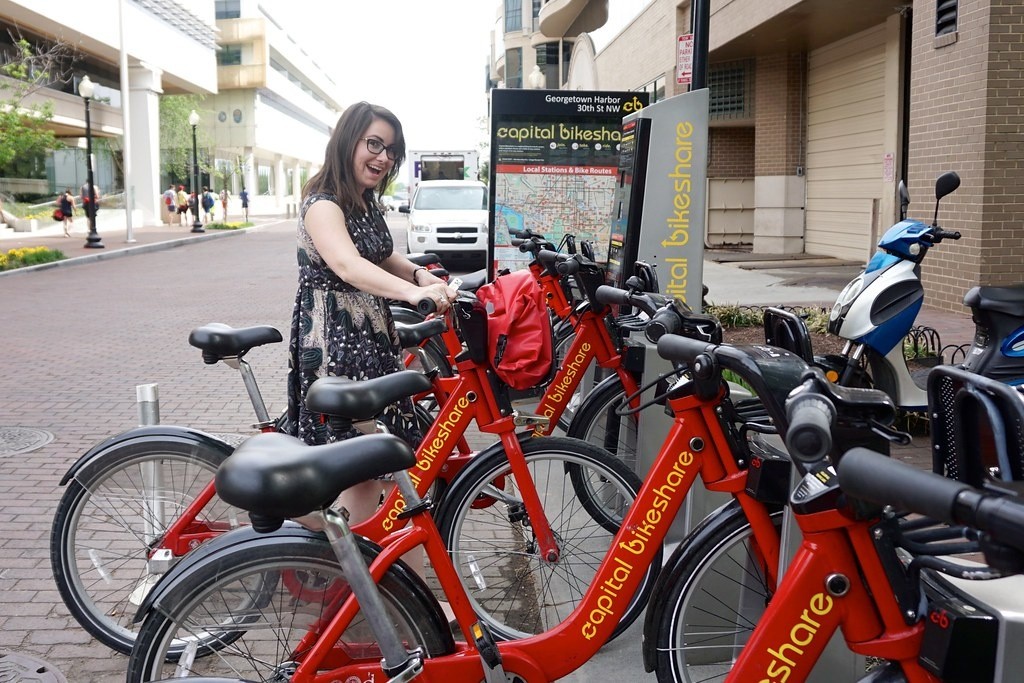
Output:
[{"left": 413, "top": 266, "right": 427, "bottom": 283}]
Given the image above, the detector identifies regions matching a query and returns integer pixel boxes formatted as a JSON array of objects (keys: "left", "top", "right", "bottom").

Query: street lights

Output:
[
  {"left": 188, "top": 108, "right": 207, "bottom": 233},
  {"left": 78, "top": 74, "right": 106, "bottom": 249}
]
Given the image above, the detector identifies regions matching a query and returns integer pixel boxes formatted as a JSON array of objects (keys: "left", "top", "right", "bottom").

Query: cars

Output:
[{"left": 380, "top": 195, "right": 396, "bottom": 212}]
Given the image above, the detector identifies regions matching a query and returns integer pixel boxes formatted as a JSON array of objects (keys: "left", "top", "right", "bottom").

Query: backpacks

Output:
[{"left": 203, "top": 193, "right": 211, "bottom": 212}]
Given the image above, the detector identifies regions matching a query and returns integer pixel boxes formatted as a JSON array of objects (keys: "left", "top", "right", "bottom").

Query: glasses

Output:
[{"left": 359, "top": 137, "right": 399, "bottom": 160}]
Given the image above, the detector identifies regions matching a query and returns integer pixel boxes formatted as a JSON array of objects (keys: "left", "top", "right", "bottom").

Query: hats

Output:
[{"left": 179, "top": 186, "right": 185, "bottom": 190}]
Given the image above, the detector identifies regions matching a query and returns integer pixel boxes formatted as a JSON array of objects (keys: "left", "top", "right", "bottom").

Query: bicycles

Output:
[{"left": 44, "top": 227, "right": 1024, "bottom": 683}]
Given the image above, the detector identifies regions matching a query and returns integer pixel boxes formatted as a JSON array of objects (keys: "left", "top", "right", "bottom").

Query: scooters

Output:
[{"left": 812, "top": 171, "right": 1024, "bottom": 427}]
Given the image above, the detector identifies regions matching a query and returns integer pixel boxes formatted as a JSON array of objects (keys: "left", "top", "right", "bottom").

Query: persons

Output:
[
  {"left": 80, "top": 180, "right": 99, "bottom": 232},
  {"left": 56, "top": 186, "right": 77, "bottom": 237},
  {"left": 163, "top": 183, "right": 232, "bottom": 227},
  {"left": 238, "top": 188, "right": 251, "bottom": 223},
  {"left": 288, "top": 100, "right": 461, "bottom": 634}
]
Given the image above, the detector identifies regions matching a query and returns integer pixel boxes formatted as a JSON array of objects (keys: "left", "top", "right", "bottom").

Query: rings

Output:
[{"left": 441, "top": 298, "right": 447, "bottom": 302}]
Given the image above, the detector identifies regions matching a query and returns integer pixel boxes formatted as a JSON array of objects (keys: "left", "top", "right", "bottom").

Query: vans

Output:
[{"left": 400, "top": 179, "right": 490, "bottom": 270}]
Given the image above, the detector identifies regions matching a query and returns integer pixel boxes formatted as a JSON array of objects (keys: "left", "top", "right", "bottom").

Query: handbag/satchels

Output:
[
  {"left": 166, "top": 197, "right": 171, "bottom": 204},
  {"left": 475, "top": 269, "right": 553, "bottom": 390},
  {"left": 53, "top": 209, "right": 64, "bottom": 221}
]
